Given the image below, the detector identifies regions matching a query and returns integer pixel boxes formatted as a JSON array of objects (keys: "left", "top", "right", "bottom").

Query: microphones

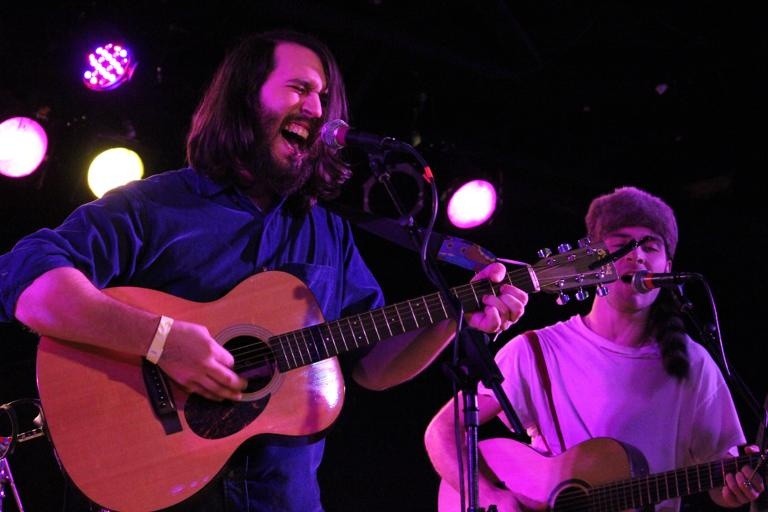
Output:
[
  {"left": 630, "top": 270, "right": 704, "bottom": 295},
  {"left": 321, "top": 119, "right": 434, "bottom": 182}
]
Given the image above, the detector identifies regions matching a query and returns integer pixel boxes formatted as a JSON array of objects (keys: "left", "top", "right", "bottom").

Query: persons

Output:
[
  {"left": 422, "top": 185, "right": 767, "bottom": 512},
  {"left": 0, "top": 29, "right": 529, "bottom": 512}
]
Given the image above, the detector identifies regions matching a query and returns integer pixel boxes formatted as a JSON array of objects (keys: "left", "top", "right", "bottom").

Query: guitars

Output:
[
  {"left": 437, "top": 435, "right": 768, "bottom": 512},
  {"left": 34, "top": 235, "right": 620, "bottom": 512}
]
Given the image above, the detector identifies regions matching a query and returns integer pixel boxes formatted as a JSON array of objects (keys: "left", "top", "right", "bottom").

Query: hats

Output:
[{"left": 586, "top": 187, "right": 680, "bottom": 259}]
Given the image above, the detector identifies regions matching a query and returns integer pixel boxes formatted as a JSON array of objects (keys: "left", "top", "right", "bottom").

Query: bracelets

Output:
[{"left": 144, "top": 314, "right": 175, "bottom": 364}]
